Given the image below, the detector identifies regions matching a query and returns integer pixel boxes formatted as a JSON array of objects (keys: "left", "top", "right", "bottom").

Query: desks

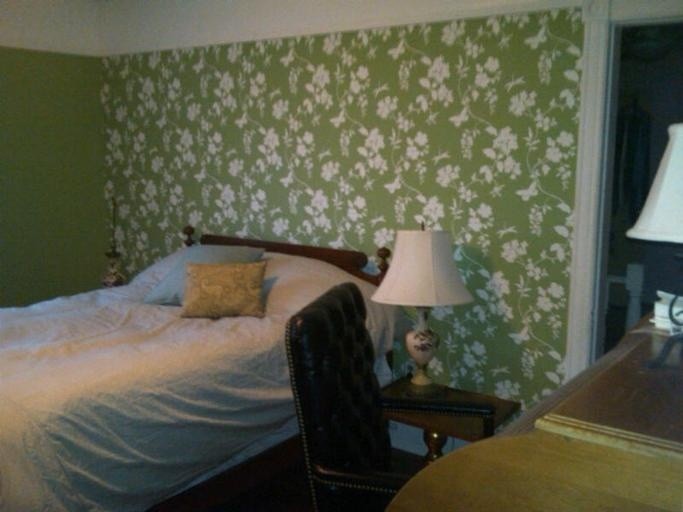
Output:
[
  {"left": 387, "top": 310, "right": 682, "bottom": 511},
  {"left": 380, "top": 375, "right": 522, "bottom": 472}
]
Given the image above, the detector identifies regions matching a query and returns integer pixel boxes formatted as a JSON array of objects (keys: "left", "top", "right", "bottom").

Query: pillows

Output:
[{"left": 122, "top": 240, "right": 269, "bottom": 321}]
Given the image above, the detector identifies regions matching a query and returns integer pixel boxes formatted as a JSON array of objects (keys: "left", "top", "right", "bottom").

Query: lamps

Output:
[
  {"left": 368, "top": 218, "right": 478, "bottom": 387},
  {"left": 624, "top": 118, "right": 682, "bottom": 371}
]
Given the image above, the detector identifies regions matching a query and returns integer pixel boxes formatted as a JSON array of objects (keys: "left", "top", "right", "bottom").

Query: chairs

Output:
[{"left": 283, "top": 282, "right": 495, "bottom": 510}]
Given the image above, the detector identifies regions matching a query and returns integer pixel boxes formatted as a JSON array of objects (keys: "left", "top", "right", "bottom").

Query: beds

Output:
[{"left": 0, "top": 225, "right": 392, "bottom": 512}]
